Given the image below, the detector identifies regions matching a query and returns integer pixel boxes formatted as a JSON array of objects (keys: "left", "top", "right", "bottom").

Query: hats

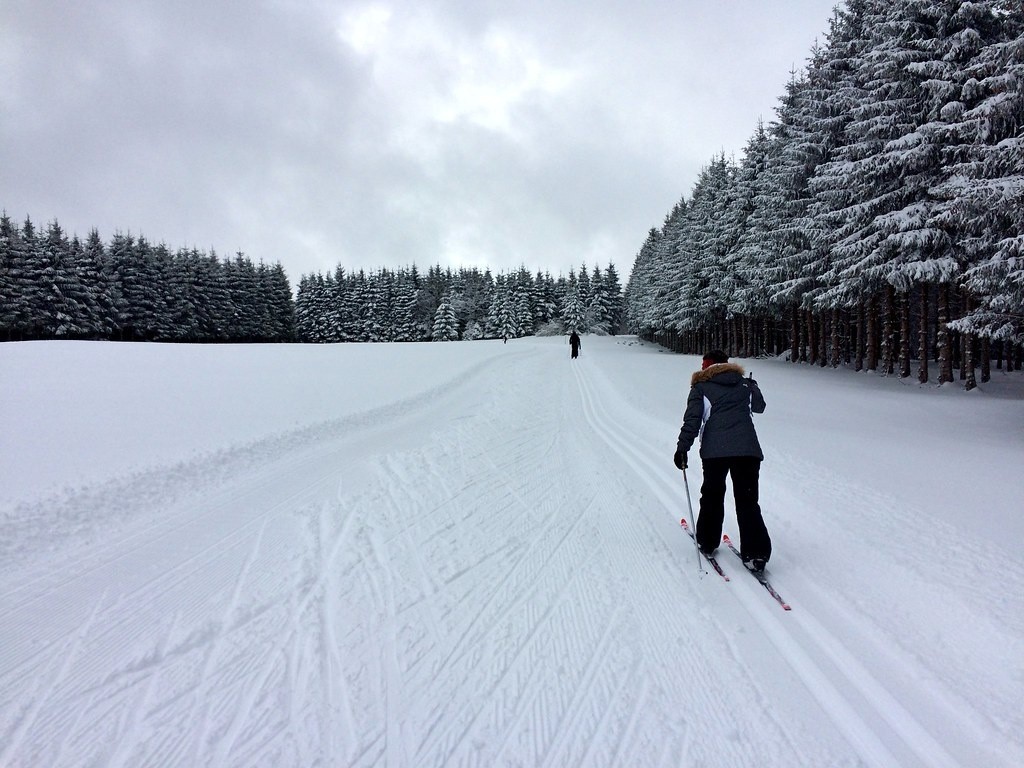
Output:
[{"left": 702, "top": 349, "right": 729, "bottom": 370}]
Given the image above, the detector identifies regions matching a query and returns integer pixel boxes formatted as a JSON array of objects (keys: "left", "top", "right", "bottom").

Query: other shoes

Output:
[
  {"left": 745, "top": 559, "right": 765, "bottom": 572},
  {"left": 700, "top": 541, "right": 715, "bottom": 555}
]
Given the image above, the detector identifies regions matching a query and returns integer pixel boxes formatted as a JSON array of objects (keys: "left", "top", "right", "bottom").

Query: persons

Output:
[
  {"left": 504, "top": 336, "right": 507, "bottom": 344},
  {"left": 674, "top": 348, "right": 772, "bottom": 573},
  {"left": 569, "top": 332, "right": 580, "bottom": 359}
]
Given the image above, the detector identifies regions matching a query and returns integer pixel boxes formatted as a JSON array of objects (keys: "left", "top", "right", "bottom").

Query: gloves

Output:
[
  {"left": 745, "top": 376, "right": 758, "bottom": 386},
  {"left": 674, "top": 450, "right": 689, "bottom": 469}
]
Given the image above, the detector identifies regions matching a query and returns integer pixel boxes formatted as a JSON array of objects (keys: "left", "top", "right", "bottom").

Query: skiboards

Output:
[
  {"left": 681, "top": 516, "right": 794, "bottom": 611},
  {"left": 570, "top": 355, "right": 579, "bottom": 360}
]
